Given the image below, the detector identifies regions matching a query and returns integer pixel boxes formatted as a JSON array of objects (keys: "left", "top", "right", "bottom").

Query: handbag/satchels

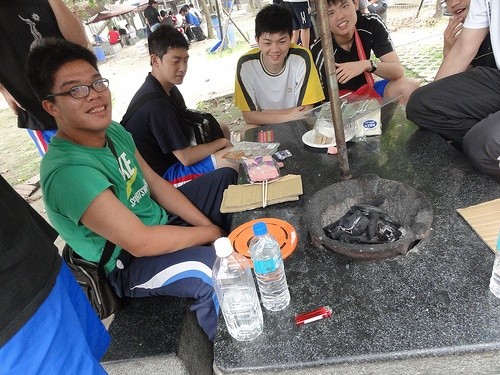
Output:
[
  {"left": 65, "top": 257, "right": 118, "bottom": 321},
  {"left": 192, "top": 112, "right": 225, "bottom": 151}
]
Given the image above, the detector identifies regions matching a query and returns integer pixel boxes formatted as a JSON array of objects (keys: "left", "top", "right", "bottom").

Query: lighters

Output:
[{"left": 295, "top": 305, "right": 332, "bottom": 325}]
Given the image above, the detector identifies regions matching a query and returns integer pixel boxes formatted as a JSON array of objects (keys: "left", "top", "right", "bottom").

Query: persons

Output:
[
  {"left": 405, "top": 0, "right": 500, "bottom": 181},
  {"left": 25, "top": 41, "right": 238, "bottom": 345},
  {"left": 93, "top": 34, "right": 103, "bottom": 43},
  {"left": 125, "top": 23, "right": 136, "bottom": 45},
  {"left": 444, "top": 0, "right": 497, "bottom": 70},
  {"left": 357, "top": 0, "right": 388, "bottom": 23},
  {"left": 144, "top": 0, "right": 202, "bottom": 43},
  {"left": 0, "top": 0, "right": 88, "bottom": 154},
  {"left": 310, "top": 0, "right": 420, "bottom": 106},
  {"left": 273, "top": 0, "right": 319, "bottom": 50},
  {"left": 234, "top": 3, "right": 326, "bottom": 142},
  {"left": 118, "top": 28, "right": 126, "bottom": 36},
  {"left": 119, "top": 24, "right": 240, "bottom": 187},
  {"left": 107, "top": 27, "right": 122, "bottom": 47},
  {"left": 0, "top": 176, "right": 111, "bottom": 375}
]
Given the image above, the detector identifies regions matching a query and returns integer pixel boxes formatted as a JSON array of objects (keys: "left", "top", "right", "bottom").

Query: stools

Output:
[
  {"left": 107, "top": 43, "right": 122, "bottom": 54},
  {"left": 127, "top": 36, "right": 140, "bottom": 44}
]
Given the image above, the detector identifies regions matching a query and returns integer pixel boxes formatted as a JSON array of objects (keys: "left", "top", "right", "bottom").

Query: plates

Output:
[
  {"left": 301, "top": 129, "right": 354, "bottom": 149},
  {"left": 227, "top": 220, "right": 297, "bottom": 267}
]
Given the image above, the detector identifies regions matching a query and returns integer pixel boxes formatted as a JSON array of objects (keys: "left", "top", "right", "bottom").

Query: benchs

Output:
[{"left": 100, "top": 299, "right": 212, "bottom": 375}]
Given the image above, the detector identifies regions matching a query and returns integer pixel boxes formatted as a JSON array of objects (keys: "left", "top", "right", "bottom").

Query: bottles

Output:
[
  {"left": 489, "top": 223, "right": 500, "bottom": 300},
  {"left": 248, "top": 222, "right": 291, "bottom": 311},
  {"left": 211, "top": 236, "right": 264, "bottom": 342}
]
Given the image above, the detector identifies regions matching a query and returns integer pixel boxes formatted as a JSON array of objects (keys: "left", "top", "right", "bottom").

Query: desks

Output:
[{"left": 213, "top": 103, "right": 500, "bottom": 375}]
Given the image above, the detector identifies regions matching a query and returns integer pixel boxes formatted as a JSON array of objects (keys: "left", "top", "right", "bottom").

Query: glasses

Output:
[{"left": 47, "top": 78, "right": 109, "bottom": 99}]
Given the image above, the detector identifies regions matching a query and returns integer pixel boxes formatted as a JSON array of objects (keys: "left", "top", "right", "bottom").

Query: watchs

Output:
[{"left": 366, "top": 60, "right": 377, "bottom": 73}]
